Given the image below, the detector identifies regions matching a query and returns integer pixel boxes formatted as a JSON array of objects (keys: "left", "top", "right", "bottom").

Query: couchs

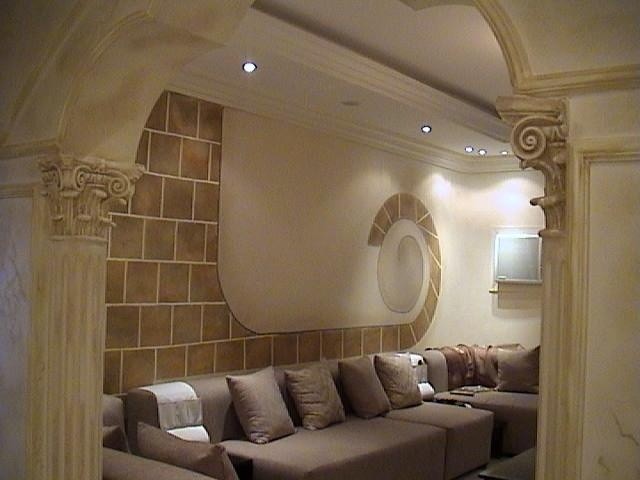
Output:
[{"left": 103, "top": 344, "right": 540, "bottom": 479}]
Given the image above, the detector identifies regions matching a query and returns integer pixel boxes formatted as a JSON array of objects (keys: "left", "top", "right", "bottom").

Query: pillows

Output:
[
  {"left": 338, "top": 355, "right": 392, "bottom": 420},
  {"left": 495, "top": 346, "right": 540, "bottom": 392},
  {"left": 375, "top": 352, "right": 423, "bottom": 410},
  {"left": 285, "top": 362, "right": 346, "bottom": 430},
  {"left": 226, "top": 365, "right": 297, "bottom": 443}
]
